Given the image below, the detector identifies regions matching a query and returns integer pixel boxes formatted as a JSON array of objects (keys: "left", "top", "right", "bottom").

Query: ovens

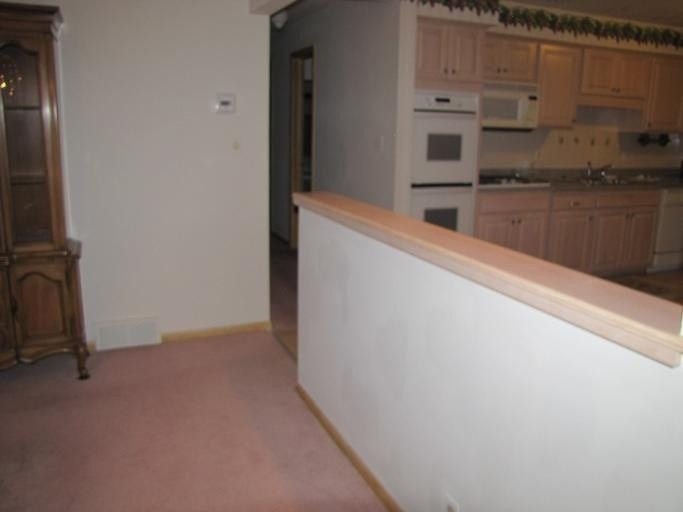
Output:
[{"left": 411, "top": 91, "right": 476, "bottom": 237}]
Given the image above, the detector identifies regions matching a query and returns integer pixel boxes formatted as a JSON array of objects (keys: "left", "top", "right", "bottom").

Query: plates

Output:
[{"left": 628, "top": 176, "right": 660, "bottom": 184}]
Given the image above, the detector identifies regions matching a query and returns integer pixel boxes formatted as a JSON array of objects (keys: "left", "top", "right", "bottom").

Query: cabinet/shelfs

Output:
[
  {"left": 577, "top": 47, "right": 652, "bottom": 111},
  {"left": 481, "top": 34, "right": 537, "bottom": 83},
  {"left": 617, "top": 54, "right": 682, "bottom": 135},
  {"left": 534, "top": 44, "right": 579, "bottom": 127},
  {"left": 548, "top": 189, "right": 593, "bottom": 276},
  {"left": 472, "top": 188, "right": 550, "bottom": 259},
  {"left": 413, "top": 14, "right": 484, "bottom": 94},
  {"left": 0, "top": 0, "right": 90, "bottom": 385},
  {"left": 592, "top": 191, "right": 660, "bottom": 277}
]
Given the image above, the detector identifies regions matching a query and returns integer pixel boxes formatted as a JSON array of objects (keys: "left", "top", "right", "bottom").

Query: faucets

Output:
[{"left": 586, "top": 159, "right": 612, "bottom": 180}]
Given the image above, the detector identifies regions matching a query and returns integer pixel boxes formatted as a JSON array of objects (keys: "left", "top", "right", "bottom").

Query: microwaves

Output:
[{"left": 478, "top": 81, "right": 539, "bottom": 131}]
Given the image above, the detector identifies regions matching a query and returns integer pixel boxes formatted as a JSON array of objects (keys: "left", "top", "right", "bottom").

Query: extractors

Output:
[{"left": 575, "top": 107, "right": 642, "bottom": 130}]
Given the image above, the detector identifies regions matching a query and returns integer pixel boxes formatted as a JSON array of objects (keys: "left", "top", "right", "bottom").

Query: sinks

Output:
[{"left": 573, "top": 169, "right": 626, "bottom": 189}]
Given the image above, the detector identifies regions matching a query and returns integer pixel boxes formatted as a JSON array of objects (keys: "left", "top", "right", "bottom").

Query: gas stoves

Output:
[{"left": 477, "top": 172, "right": 550, "bottom": 189}]
